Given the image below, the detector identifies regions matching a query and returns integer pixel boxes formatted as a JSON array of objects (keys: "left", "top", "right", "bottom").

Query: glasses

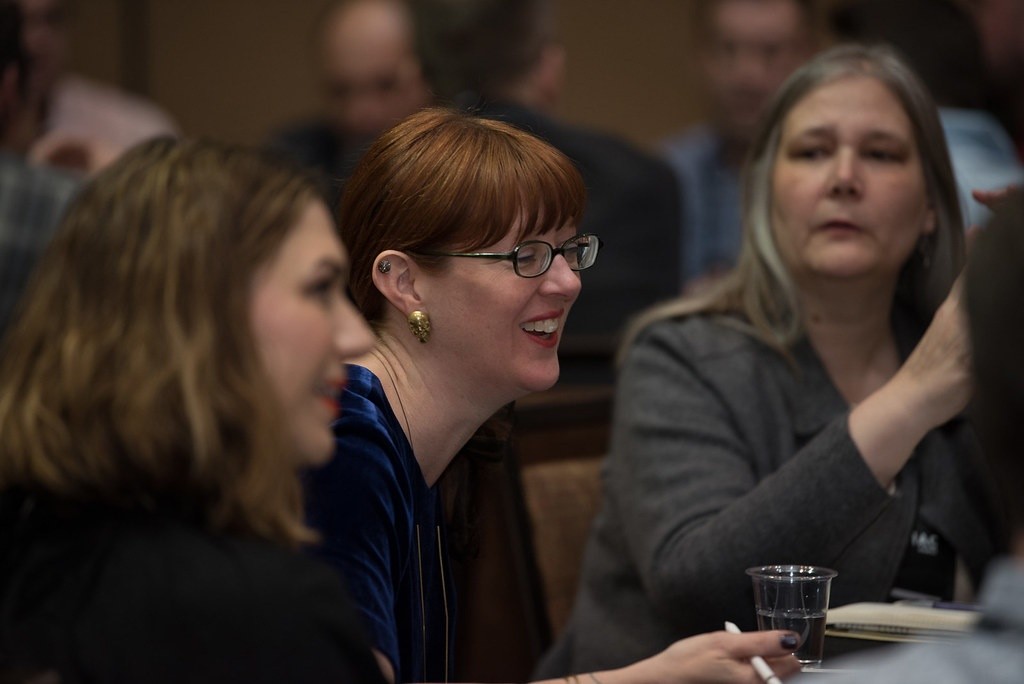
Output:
[{"left": 407, "top": 232, "right": 605, "bottom": 278}]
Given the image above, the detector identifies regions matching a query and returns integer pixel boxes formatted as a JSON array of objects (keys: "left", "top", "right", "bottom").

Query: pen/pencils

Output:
[{"left": 724, "top": 620, "right": 781, "bottom": 684}]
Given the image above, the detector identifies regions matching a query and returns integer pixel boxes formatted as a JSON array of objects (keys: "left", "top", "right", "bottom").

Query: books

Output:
[{"left": 826, "top": 602, "right": 980, "bottom": 643}]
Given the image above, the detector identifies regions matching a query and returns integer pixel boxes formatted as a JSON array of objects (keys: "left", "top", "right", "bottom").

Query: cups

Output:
[{"left": 746, "top": 565, "right": 838, "bottom": 670}]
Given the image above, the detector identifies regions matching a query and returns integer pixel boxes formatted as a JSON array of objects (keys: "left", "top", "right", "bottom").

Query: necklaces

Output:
[{"left": 370, "top": 351, "right": 413, "bottom": 452}]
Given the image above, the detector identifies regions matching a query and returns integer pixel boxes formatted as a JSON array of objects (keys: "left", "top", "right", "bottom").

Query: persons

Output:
[
  {"left": 303, "top": 111, "right": 800, "bottom": 683},
  {"left": 527, "top": 41, "right": 1006, "bottom": 684},
  {"left": 0, "top": 0, "right": 176, "bottom": 319},
  {"left": 270, "top": 0, "right": 682, "bottom": 383},
  {"left": 0, "top": 138, "right": 387, "bottom": 684},
  {"left": 805, "top": 186, "right": 1024, "bottom": 684},
  {"left": 672, "top": 0, "right": 1023, "bottom": 276}
]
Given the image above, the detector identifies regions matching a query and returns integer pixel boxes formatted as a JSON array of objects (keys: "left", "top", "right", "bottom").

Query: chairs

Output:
[{"left": 502, "top": 441, "right": 607, "bottom": 663}]
